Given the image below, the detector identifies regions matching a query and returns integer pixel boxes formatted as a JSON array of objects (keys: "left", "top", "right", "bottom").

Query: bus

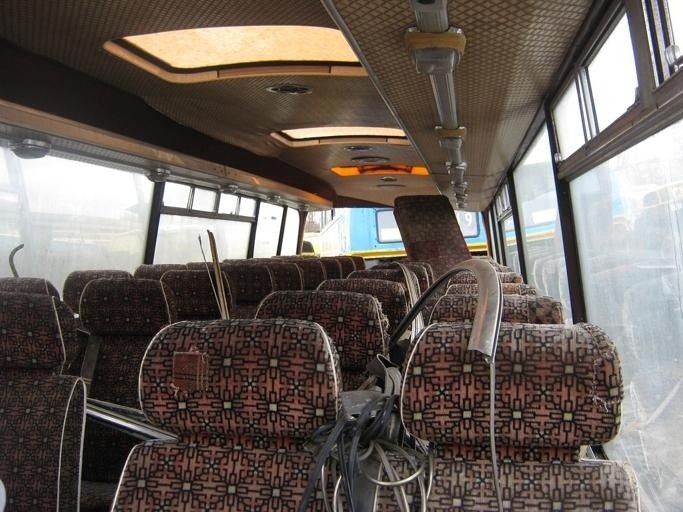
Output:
[{"left": 302, "top": 157, "right": 626, "bottom": 261}]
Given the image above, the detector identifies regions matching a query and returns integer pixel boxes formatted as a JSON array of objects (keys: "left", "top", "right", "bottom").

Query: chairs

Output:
[
  {"left": 2, "top": 255, "right": 564, "bottom": 404},
  {"left": 71, "top": 280, "right": 177, "bottom": 504},
  {"left": 1, "top": 292, "right": 86, "bottom": 506},
  {"left": 397, "top": 322, "right": 641, "bottom": 512},
  {"left": 107, "top": 321, "right": 346, "bottom": 512}
]
